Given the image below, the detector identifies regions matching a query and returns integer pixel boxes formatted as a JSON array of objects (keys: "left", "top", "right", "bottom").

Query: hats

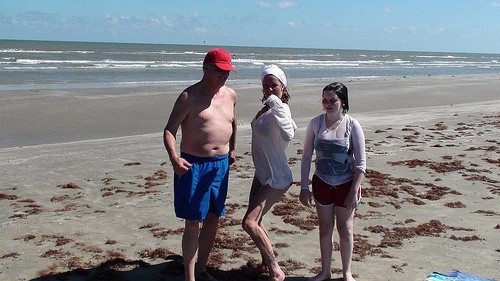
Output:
[
  {"left": 260, "top": 64, "right": 287, "bottom": 87},
  {"left": 203, "top": 48, "right": 237, "bottom": 73}
]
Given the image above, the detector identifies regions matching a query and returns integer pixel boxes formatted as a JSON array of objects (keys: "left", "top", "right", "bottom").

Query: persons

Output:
[
  {"left": 162, "top": 49, "right": 237, "bottom": 281},
  {"left": 300, "top": 82, "right": 366, "bottom": 281},
  {"left": 242, "top": 65, "right": 297, "bottom": 281}
]
